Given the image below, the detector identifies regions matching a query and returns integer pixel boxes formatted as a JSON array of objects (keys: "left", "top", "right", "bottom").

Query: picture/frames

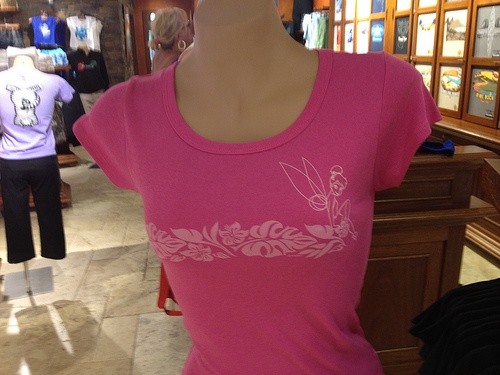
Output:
[{"left": 328, "top": 0, "right": 500, "bottom": 129}]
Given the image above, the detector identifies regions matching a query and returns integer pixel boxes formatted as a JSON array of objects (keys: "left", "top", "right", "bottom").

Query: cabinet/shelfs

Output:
[{"left": 356, "top": 136, "right": 498, "bottom": 365}]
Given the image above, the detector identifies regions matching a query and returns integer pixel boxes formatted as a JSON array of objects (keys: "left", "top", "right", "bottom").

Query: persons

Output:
[
  {"left": 148, "top": 7, "right": 191, "bottom": 72},
  {"left": 72, "top": 0, "right": 443, "bottom": 374},
  {"left": 1, "top": 55, "right": 76, "bottom": 264}
]
